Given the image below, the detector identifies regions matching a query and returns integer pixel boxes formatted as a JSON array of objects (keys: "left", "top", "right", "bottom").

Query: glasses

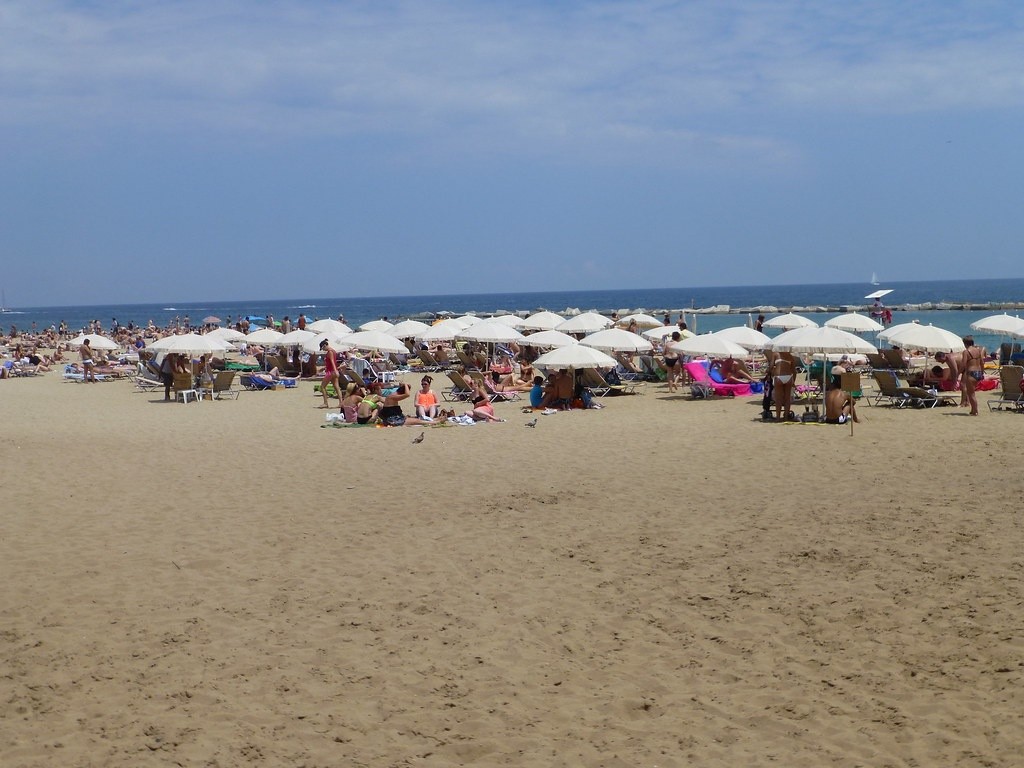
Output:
[
  {"left": 470, "top": 381, "right": 475, "bottom": 386},
  {"left": 420, "top": 383, "right": 428, "bottom": 385}
]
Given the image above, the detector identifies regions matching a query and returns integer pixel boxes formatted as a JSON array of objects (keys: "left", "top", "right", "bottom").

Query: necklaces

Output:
[{"left": 423, "top": 390, "right": 429, "bottom": 394}]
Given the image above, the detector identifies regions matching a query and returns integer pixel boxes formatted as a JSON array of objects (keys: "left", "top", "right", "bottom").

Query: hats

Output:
[
  {"left": 362, "top": 369, "right": 370, "bottom": 374},
  {"left": 344, "top": 382, "right": 357, "bottom": 397}
]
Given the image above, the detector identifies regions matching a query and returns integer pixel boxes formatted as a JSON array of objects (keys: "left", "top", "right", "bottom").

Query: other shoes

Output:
[
  {"left": 319, "top": 404, "right": 328, "bottom": 408},
  {"left": 592, "top": 403, "right": 606, "bottom": 409}
]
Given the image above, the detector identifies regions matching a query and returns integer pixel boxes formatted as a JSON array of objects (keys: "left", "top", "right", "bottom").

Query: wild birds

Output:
[
  {"left": 412, "top": 431, "right": 425, "bottom": 444},
  {"left": 524, "top": 418, "right": 537, "bottom": 428}
]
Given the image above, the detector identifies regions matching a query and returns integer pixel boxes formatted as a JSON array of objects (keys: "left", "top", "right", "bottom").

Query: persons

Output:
[
  {"left": 651, "top": 313, "right": 997, "bottom": 425},
  {"left": 868, "top": 298, "right": 887, "bottom": 323},
  {"left": 0, "top": 312, "right": 638, "bottom": 426}
]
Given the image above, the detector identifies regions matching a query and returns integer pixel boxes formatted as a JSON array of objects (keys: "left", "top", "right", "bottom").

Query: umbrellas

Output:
[
  {"left": 146, "top": 310, "right": 663, "bottom": 400},
  {"left": 642, "top": 312, "right": 1024, "bottom": 356},
  {"left": 67, "top": 334, "right": 118, "bottom": 350}
]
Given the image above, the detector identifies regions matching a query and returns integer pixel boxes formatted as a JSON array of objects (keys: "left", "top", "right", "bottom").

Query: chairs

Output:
[{"left": 130, "top": 342, "right": 1024, "bottom": 413}]
